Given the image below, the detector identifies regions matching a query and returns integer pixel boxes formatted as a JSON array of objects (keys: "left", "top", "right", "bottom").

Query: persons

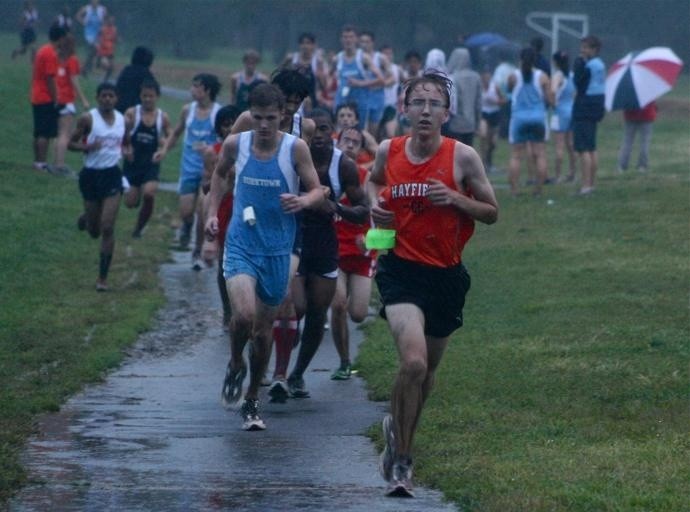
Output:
[
  {"left": 368, "top": 78, "right": 499, "bottom": 497},
  {"left": 12, "top": 1, "right": 118, "bottom": 83},
  {"left": 402, "top": 34, "right": 605, "bottom": 197},
  {"left": 66, "top": 46, "right": 174, "bottom": 291},
  {"left": 151, "top": 24, "right": 404, "bottom": 430},
  {"left": 616, "top": 100, "right": 657, "bottom": 174},
  {"left": 32, "top": 23, "right": 87, "bottom": 181}
]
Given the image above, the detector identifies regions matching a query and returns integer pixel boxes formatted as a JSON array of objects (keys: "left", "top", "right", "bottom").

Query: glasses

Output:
[{"left": 407, "top": 100, "right": 449, "bottom": 113}]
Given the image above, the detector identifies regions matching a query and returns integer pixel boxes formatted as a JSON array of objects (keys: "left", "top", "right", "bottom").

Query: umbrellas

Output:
[{"left": 604, "top": 45, "right": 685, "bottom": 112}]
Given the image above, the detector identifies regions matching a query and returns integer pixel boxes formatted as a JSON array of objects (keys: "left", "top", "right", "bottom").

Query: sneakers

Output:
[
  {"left": 177, "top": 216, "right": 195, "bottom": 246},
  {"left": 507, "top": 165, "right": 648, "bottom": 199},
  {"left": 190, "top": 255, "right": 204, "bottom": 271},
  {"left": 220, "top": 355, "right": 247, "bottom": 411},
  {"left": 94, "top": 277, "right": 109, "bottom": 291},
  {"left": 132, "top": 230, "right": 145, "bottom": 241},
  {"left": 239, "top": 397, "right": 266, "bottom": 430},
  {"left": 77, "top": 212, "right": 89, "bottom": 232},
  {"left": 31, "top": 159, "right": 79, "bottom": 178},
  {"left": 377, "top": 414, "right": 415, "bottom": 497},
  {"left": 288, "top": 370, "right": 310, "bottom": 399},
  {"left": 330, "top": 362, "right": 352, "bottom": 381},
  {"left": 268, "top": 378, "right": 290, "bottom": 404}
]
